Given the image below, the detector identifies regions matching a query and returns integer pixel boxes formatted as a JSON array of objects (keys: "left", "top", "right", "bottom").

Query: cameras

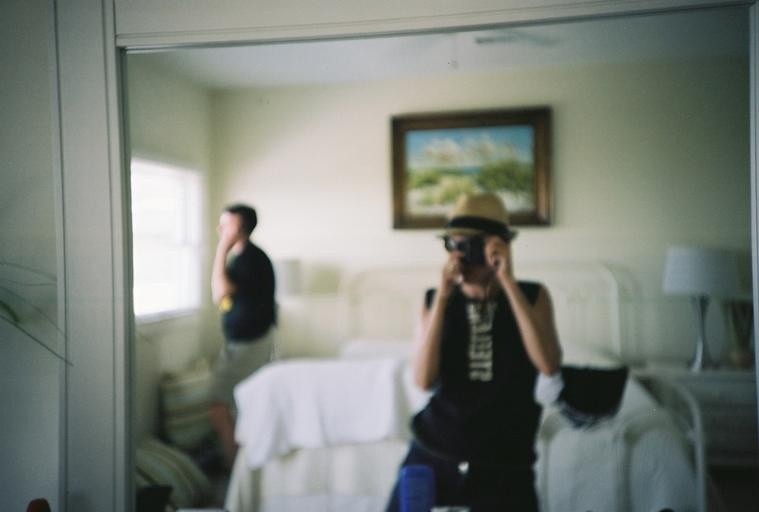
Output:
[{"left": 456, "top": 237, "right": 485, "bottom": 265}]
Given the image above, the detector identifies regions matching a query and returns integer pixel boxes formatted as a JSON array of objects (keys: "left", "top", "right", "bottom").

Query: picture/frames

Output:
[{"left": 389, "top": 104, "right": 551, "bottom": 230}]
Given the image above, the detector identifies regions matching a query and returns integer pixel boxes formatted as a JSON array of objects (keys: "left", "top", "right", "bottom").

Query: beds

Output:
[{"left": 228, "top": 258, "right": 709, "bottom": 512}]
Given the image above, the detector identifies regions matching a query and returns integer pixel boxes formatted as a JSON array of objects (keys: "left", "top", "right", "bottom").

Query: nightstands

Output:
[{"left": 649, "top": 360, "right": 759, "bottom": 470}]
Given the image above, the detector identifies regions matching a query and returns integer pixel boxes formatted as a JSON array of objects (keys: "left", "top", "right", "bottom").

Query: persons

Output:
[
  {"left": 209, "top": 205, "right": 277, "bottom": 473},
  {"left": 383, "top": 192, "right": 563, "bottom": 512}
]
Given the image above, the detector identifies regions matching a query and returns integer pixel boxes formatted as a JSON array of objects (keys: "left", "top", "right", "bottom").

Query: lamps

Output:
[{"left": 661, "top": 244, "right": 726, "bottom": 369}]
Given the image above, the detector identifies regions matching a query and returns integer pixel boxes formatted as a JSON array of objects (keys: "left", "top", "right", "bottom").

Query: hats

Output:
[{"left": 436, "top": 192, "right": 515, "bottom": 240}]
